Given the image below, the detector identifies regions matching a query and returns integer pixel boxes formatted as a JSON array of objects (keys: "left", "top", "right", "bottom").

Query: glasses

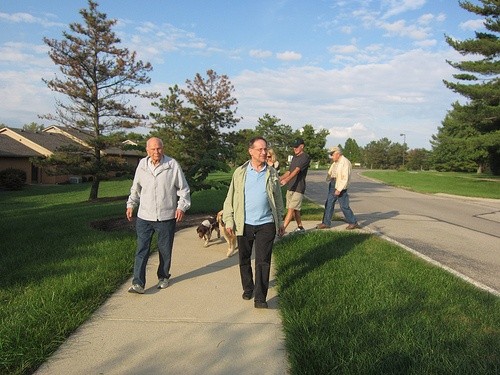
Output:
[
  {"left": 267, "top": 156, "right": 271, "bottom": 158},
  {"left": 252, "top": 148, "right": 267, "bottom": 152}
]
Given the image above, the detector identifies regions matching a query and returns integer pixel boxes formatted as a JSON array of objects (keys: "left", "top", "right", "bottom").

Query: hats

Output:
[
  {"left": 329, "top": 146, "right": 338, "bottom": 154},
  {"left": 290, "top": 137, "right": 304, "bottom": 148}
]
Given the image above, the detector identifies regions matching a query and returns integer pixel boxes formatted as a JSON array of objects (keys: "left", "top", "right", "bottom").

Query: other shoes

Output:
[
  {"left": 255, "top": 300, "right": 268, "bottom": 308},
  {"left": 295, "top": 228, "right": 305, "bottom": 232},
  {"left": 242, "top": 289, "right": 253, "bottom": 300},
  {"left": 346, "top": 223, "right": 358, "bottom": 229},
  {"left": 316, "top": 224, "right": 330, "bottom": 229}
]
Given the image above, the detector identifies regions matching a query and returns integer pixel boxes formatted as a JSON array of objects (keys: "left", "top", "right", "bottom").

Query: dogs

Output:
[
  {"left": 196, "top": 216, "right": 221, "bottom": 248},
  {"left": 215, "top": 209, "right": 237, "bottom": 257}
]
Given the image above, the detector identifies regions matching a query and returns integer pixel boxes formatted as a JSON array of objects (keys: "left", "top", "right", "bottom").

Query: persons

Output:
[
  {"left": 316, "top": 147, "right": 358, "bottom": 230},
  {"left": 278, "top": 137, "right": 310, "bottom": 232},
  {"left": 126, "top": 137, "right": 191, "bottom": 293},
  {"left": 265, "top": 148, "right": 280, "bottom": 176},
  {"left": 223, "top": 137, "right": 285, "bottom": 309}
]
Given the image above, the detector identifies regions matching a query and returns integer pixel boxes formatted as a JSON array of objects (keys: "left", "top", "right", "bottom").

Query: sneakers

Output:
[
  {"left": 128, "top": 284, "right": 144, "bottom": 294},
  {"left": 157, "top": 278, "right": 169, "bottom": 289}
]
